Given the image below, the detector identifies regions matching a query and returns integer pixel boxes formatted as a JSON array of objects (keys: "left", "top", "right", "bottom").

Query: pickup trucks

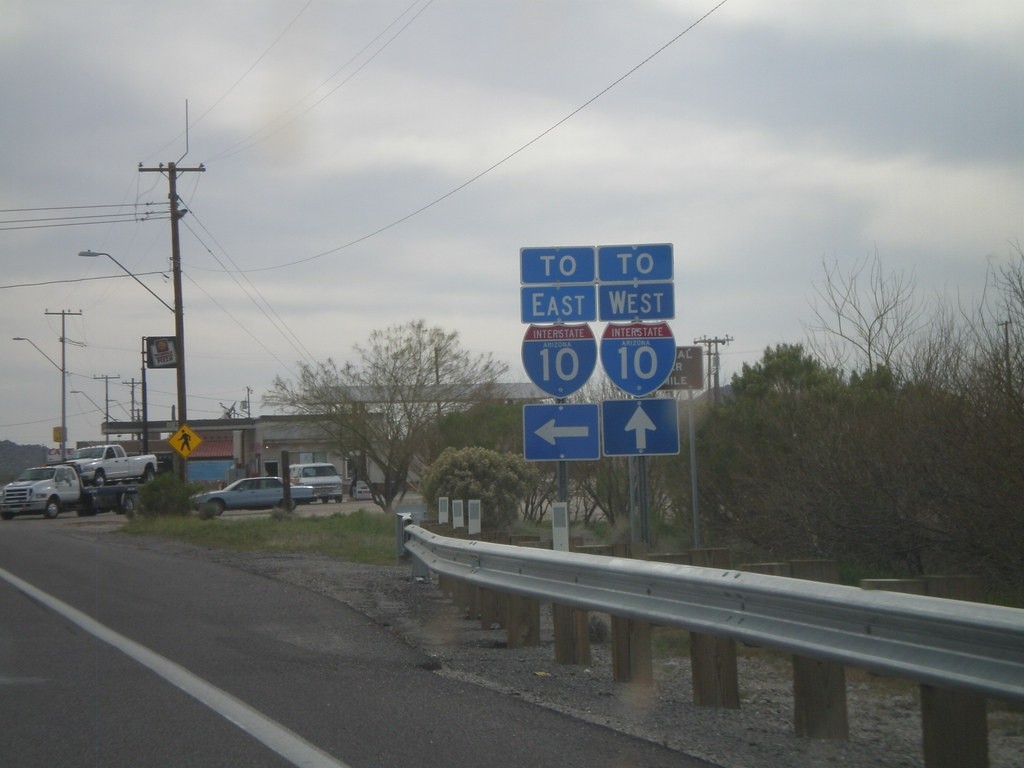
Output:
[{"left": 63, "top": 444, "right": 158, "bottom": 487}]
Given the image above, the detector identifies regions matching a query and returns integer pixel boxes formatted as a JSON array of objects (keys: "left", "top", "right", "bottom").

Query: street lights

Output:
[
  {"left": 78, "top": 248, "right": 186, "bottom": 488},
  {"left": 12, "top": 337, "right": 67, "bottom": 461}
]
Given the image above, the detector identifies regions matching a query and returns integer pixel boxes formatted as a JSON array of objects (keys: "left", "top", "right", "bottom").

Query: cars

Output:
[
  {"left": 188, "top": 477, "right": 315, "bottom": 517},
  {"left": 352, "top": 479, "right": 374, "bottom": 501}
]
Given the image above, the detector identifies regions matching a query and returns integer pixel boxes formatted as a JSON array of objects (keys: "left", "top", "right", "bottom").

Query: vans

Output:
[{"left": 288, "top": 463, "right": 344, "bottom": 504}]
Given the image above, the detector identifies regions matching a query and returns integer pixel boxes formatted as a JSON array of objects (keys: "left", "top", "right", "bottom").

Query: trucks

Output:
[{"left": 0, "top": 462, "right": 140, "bottom": 520}]
